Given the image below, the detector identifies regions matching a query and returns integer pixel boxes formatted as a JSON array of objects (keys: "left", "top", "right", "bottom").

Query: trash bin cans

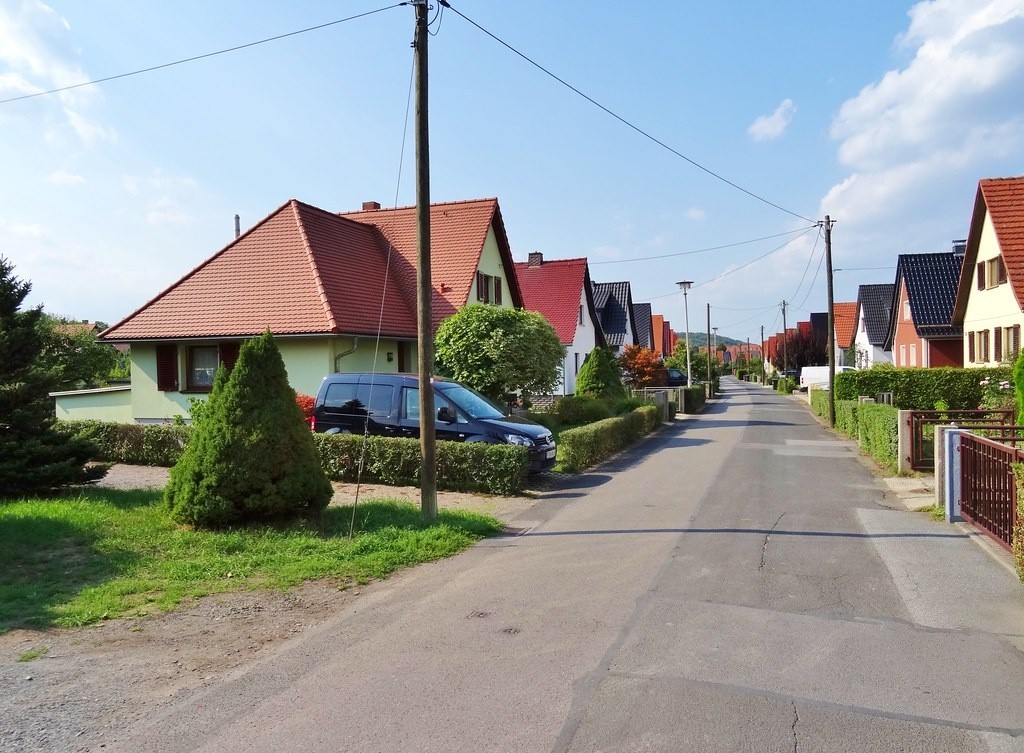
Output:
[
  {"left": 743, "top": 375, "right": 749, "bottom": 382},
  {"left": 772, "top": 380, "right": 778, "bottom": 390}
]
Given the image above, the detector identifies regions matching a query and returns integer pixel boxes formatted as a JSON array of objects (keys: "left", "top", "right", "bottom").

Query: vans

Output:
[
  {"left": 780, "top": 371, "right": 800, "bottom": 384},
  {"left": 310, "top": 373, "right": 558, "bottom": 476},
  {"left": 666, "top": 369, "right": 697, "bottom": 387},
  {"left": 800, "top": 365, "right": 859, "bottom": 391}
]
